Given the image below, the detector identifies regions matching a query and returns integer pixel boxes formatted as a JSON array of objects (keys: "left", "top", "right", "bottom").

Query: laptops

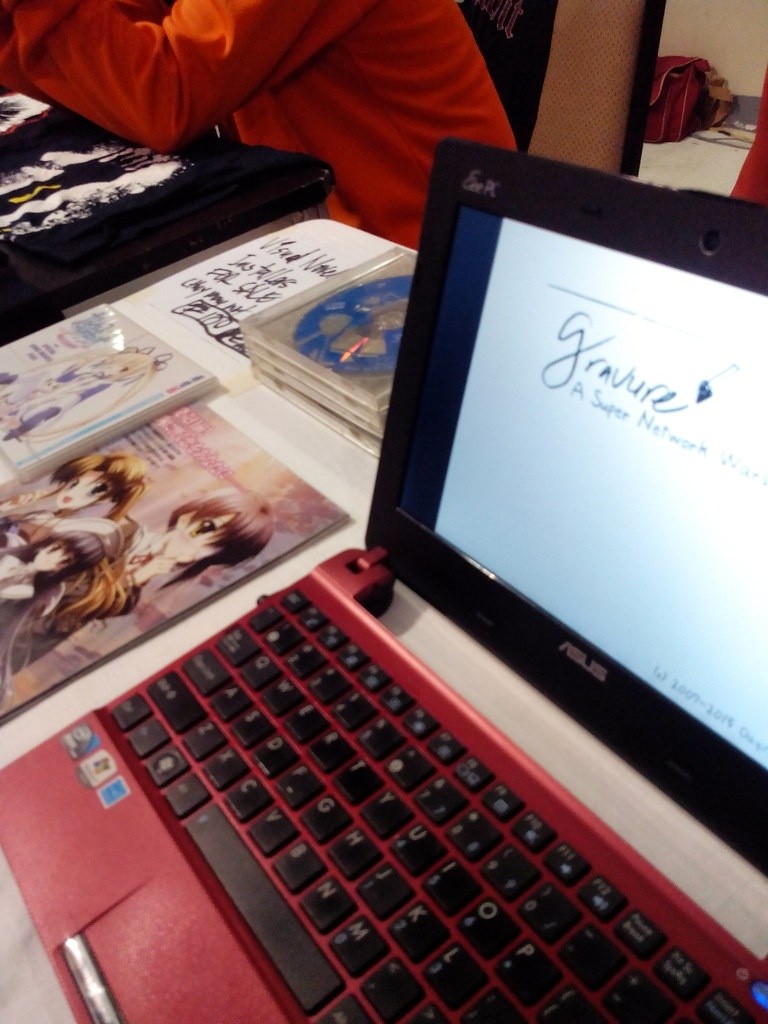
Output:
[{"left": 0, "top": 136, "right": 768, "bottom": 1024}]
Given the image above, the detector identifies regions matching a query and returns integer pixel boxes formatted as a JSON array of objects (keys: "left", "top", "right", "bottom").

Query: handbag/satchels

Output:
[{"left": 643, "top": 56, "right": 734, "bottom": 144}]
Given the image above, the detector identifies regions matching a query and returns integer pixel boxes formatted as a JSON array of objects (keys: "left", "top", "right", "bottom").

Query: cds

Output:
[{"left": 293, "top": 273, "right": 414, "bottom": 373}]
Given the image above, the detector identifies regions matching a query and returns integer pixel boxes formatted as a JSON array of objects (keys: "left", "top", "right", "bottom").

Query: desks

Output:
[
  {"left": 0, "top": 86, "right": 335, "bottom": 348},
  {"left": 0, "top": 219, "right": 420, "bottom": 1024}
]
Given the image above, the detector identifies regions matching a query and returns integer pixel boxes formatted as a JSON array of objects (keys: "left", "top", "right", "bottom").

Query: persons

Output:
[{"left": 0, "top": 0, "right": 519, "bottom": 251}]
